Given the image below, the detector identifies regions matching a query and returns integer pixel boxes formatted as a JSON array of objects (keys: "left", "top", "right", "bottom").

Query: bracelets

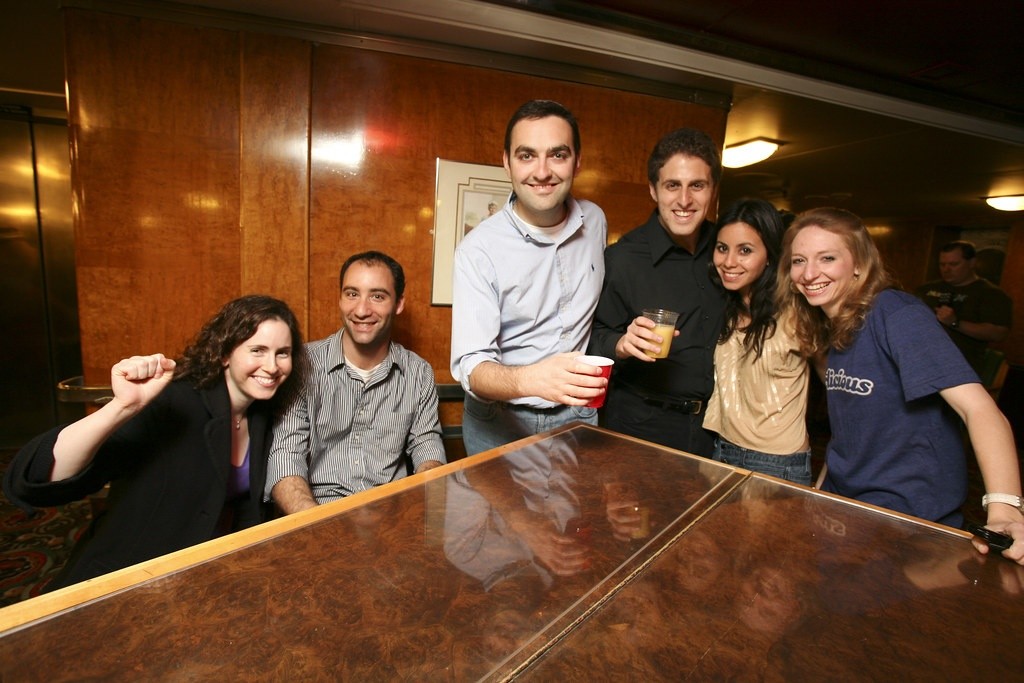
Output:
[
  {"left": 950, "top": 318, "right": 962, "bottom": 331},
  {"left": 982, "top": 493, "right": 1024, "bottom": 517}
]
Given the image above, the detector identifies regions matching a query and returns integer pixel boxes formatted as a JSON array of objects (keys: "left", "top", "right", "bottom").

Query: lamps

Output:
[
  {"left": 721, "top": 102, "right": 786, "bottom": 170},
  {"left": 976, "top": 175, "right": 1024, "bottom": 212}
]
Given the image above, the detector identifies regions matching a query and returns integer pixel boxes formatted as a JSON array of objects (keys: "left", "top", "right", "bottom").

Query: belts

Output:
[{"left": 619, "top": 384, "right": 709, "bottom": 415}]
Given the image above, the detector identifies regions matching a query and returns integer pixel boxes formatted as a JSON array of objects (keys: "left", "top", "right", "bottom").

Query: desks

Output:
[{"left": 0, "top": 421, "right": 1024, "bottom": 683}]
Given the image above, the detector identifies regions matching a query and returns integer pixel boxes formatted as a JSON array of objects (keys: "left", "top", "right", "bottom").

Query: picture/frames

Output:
[{"left": 430, "top": 158, "right": 512, "bottom": 306}]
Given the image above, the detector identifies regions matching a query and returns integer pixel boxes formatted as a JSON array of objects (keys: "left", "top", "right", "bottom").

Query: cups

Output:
[
  {"left": 578, "top": 356, "right": 614, "bottom": 408},
  {"left": 643, "top": 308, "right": 679, "bottom": 358}
]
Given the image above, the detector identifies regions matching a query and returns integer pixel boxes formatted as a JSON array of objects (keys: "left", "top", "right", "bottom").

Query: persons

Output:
[
  {"left": 910, "top": 240, "right": 1015, "bottom": 388},
  {"left": 771, "top": 205, "right": 1024, "bottom": 561},
  {"left": 698, "top": 198, "right": 811, "bottom": 489},
  {"left": 1, "top": 294, "right": 314, "bottom": 593},
  {"left": 264, "top": 251, "right": 448, "bottom": 515},
  {"left": 450, "top": 98, "right": 608, "bottom": 455},
  {"left": 601, "top": 439, "right": 1024, "bottom": 635},
  {"left": 438, "top": 429, "right": 582, "bottom": 678},
  {"left": 586, "top": 129, "right": 741, "bottom": 458}
]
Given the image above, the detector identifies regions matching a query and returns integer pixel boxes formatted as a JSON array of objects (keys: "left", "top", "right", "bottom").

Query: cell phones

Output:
[{"left": 968, "top": 524, "right": 1013, "bottom": 549}]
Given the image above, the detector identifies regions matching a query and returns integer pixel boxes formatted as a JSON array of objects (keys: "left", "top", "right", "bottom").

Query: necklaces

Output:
[{"left": 230, "top": 408, "right": 247, "bottom": 433}]
